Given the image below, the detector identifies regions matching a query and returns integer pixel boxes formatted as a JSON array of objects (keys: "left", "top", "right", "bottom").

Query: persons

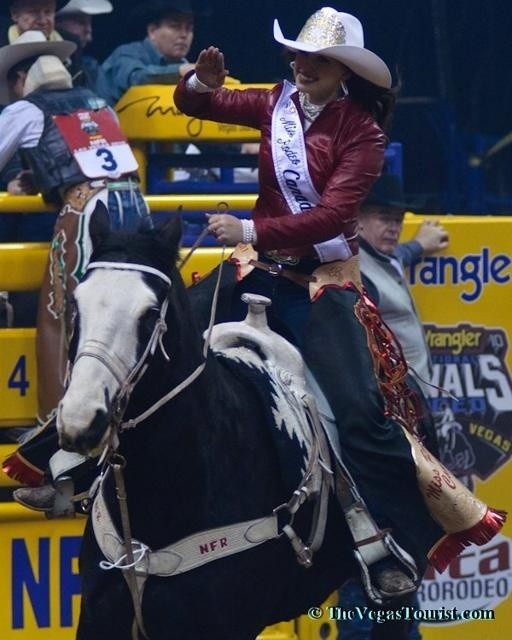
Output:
[
  {"left": 0, "top": 31, "right": 155, "bottom": 424},
  {"left": 7, "top": 0, "right": 91, "bottom": 89},
  {"left": 12, "top": 6, "right": 419, "bottom": 597},
  {"left": 356, "top": 174, "right": 449, "bottom": 463},
  {"left": 101, "top": 10, "right": 241, "bottom": 109},
  {"left": 55, "top": 0, "right": 114, "bottom": 94}
]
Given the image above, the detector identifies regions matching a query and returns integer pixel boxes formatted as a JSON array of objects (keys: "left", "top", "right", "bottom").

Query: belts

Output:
[{"left": 261, "top": 245, "right": 322, "bottom": 272}]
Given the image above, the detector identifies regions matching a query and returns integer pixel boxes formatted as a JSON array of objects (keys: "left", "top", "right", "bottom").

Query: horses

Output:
[{"left": 57, "top": 199, "right": 441, "bottom": 640}]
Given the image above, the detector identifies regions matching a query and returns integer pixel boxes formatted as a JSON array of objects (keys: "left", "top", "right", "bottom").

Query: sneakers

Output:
[
  {"left": 11, "top": 484, "right": 61, "bottom": 511},
  {"left": 373, "top": 552, "right": 416, "bottom": 598}
]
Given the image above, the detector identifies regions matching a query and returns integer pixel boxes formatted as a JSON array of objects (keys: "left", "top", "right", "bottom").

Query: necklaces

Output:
[{"left": 297, "top": 88, "right": 347, "bottom": 118}]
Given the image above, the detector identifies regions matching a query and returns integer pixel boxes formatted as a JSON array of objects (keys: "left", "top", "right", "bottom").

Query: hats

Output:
[
  {"left": 273, "top": 7, "right": 392, "bottom": 89},
  {"left": 0, "top": 1, "right": 69, "bottom": 13},
  {"left": 59, "top": 1, "right": 113, "bottom": 15},
  {"left": 1, "top": 30, "right": 78, "bottom": 79},
  {"left": 357, "top": 175, "right": 426, "bottom": 214}
]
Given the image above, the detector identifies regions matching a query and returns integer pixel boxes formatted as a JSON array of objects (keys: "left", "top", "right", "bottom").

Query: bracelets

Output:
[{"left": 244, "top": 218, "right": 254, "bottom": 242}]
